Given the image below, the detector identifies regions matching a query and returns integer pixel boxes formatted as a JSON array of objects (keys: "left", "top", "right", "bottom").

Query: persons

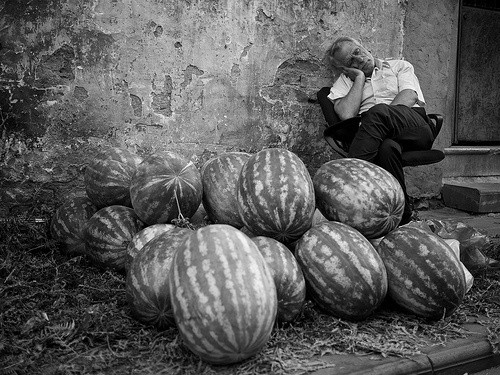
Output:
[{"left": 327, "top": 36, "right": 437, "bottom": 227}]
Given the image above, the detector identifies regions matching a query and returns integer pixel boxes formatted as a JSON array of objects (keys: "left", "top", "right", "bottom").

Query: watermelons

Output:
[{"left": 50, "top": 147, "right": 467, "bottom": 364}]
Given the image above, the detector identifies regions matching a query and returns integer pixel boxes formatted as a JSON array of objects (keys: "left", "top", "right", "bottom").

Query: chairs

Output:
[{"left": 316, "top": 87, "right": 445, "bottom": 166}]
